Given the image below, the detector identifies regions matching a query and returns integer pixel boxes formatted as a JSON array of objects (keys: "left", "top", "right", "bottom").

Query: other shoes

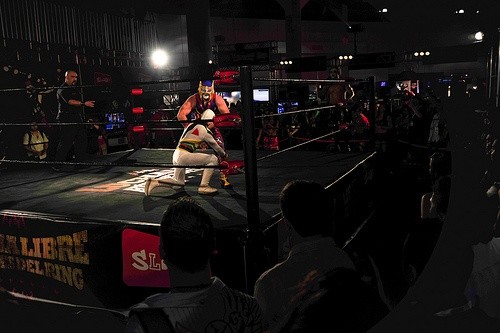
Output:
[
  {"left": 51, "top": 164, "right": 63, "bottom": 172},
  {"left": 198, "top": 185, "right": 218, "bottom": 194},
  {"left": 145, "top": 177, "right": 159, "bottom": 196}
]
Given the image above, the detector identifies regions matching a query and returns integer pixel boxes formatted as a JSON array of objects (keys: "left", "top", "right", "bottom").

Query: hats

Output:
[
  {"left": 201, "top": 109, "right": 215, "bottom": 119},
  {"left": 203, "top": 81, "right": 211, "bottom": 87}
]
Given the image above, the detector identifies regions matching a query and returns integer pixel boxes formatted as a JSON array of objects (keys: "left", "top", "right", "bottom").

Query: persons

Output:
[
  {"left": 274, "top": 268, "right": 390, "bottom": 333},
  {"left": 22, "top": 118, "right": 49, "bottom": 161},
  {"left": 224, "top": 93, "right": 396, "bottom": 154},
  {"left": 375, "top": 82, "right": 499, "bottom": 333},
  {"left": 176, "top": 80, "right": 232, "bottom": 188},
  {"left": 316, "top": 66, "right": 356, "bottom": 153},
  {"left": 85, "top": 103, "right": 184, "bottom": 156},
  {"left": 51, "top": 70, "right": 96, "bottom": 172},
  {"left": 252, "top": 180, "right": 355, "bottom": 333},
  {"left": 144, "top": 109, "right": 229, "bottom": 196},
  {"left": 126, "top": 197, "right": 270, "bottom": 333}
]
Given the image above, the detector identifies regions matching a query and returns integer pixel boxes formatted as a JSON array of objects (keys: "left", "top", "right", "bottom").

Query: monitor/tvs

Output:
[{"left": 253, "top": 89, "right": 270, "bottom": 102}]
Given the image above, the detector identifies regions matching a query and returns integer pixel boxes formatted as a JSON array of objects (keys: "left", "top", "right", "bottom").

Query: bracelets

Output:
[{"left": 81, "top": 102, "right": 85, "bottom": 106}]
